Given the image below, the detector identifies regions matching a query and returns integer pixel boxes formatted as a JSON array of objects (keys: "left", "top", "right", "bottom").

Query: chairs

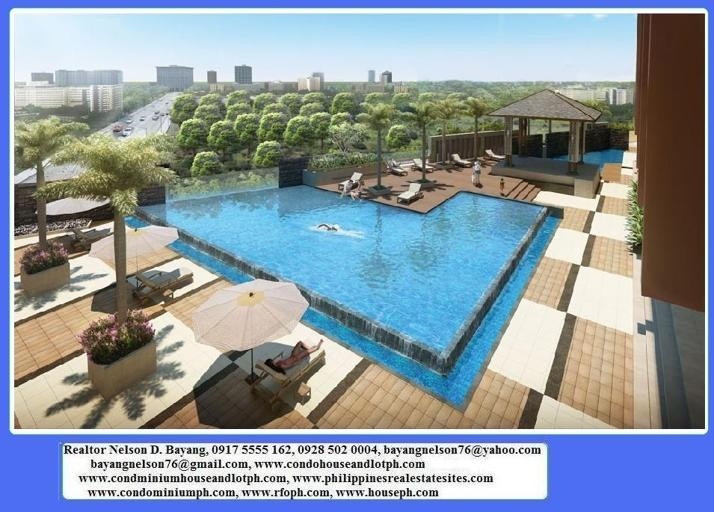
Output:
[
  {"left": 70, "top": 227, "right": 111, "bottom": 250},
  {"left": 249, "top": 343, "right": 326, "bottom": 408},
  {"left": 132, "top": 267, "right": 194, "bottom": 299},
  {"left": 336, "top": 147, "right": 506, "bottom": 206}
]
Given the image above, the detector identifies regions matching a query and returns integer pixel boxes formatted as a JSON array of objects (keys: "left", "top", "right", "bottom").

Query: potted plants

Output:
[
  {"left": 13, "top": 114, "right": 89, "bottom": 297},
  {"left": 29, "top": 131, "right": 180, "bottom": 401}
]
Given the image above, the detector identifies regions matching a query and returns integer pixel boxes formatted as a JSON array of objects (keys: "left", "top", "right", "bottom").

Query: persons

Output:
[
  {"left": 473, "top": 161, "right": 481, "bottom": 187},
  {"left": 500, "top": 177, "right": 505, "bottom": 192},
  {"left": 391, "top": 158, "right": 404, "bottom": 170},
  {"left": 265, "top": 339, "right": 323, "bottom": 374},
  {"left": 317, "top": 224, "right": 337, "bottom": 231},
  {"left": 342, "top": 179, "right": 361, "bottom": 200}
]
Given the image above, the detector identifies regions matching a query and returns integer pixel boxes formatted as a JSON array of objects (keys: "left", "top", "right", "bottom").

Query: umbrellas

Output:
[
  {"left": 89, "top": 224, "right": 181, "bottom": 291},
  {"left": 34, "top": 193, "right": 111, "bottom": 238},
  {"left": 190, "top": 278, "right": 310, "bottom": 383}
]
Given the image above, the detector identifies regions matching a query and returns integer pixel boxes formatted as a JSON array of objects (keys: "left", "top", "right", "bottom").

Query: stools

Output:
[
  {"left": 293, "top": 381, "right": 312, "bottom": 404},
  {"left": 161, "top": 289, "right": 173, "bottom": 302}
]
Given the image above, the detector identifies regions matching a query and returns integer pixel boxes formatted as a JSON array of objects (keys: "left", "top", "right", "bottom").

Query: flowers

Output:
[
  {"left": 19, "top": 239, "right": 68, "bottom": 274},
  {"left": 76, "top": 308, "right": 155, "bottom": 363}
]
